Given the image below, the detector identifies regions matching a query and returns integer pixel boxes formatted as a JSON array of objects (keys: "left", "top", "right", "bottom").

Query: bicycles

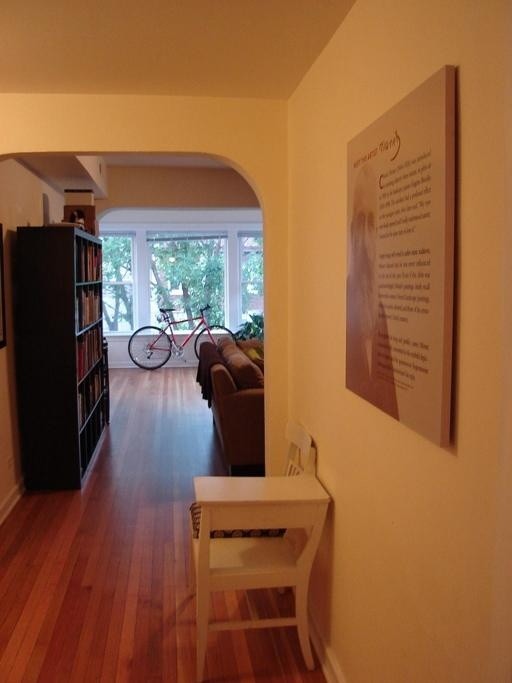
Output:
[{"left": 129, "top": 305, "right": 239, "bottom": 370}]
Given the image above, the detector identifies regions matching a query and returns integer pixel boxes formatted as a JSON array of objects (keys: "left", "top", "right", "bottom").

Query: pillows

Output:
[{"left": 236, "top": 340, "right": 264, "bottom": 374}]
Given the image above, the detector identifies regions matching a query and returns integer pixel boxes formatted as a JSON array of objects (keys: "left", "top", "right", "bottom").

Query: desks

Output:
[{"left": 193, "top": 476, "right": 331, "bottom": 683}]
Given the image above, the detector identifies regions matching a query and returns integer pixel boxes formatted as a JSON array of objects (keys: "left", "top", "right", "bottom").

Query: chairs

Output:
[{"left": 190, "top": 422, "right": 319, "bottom": 594}]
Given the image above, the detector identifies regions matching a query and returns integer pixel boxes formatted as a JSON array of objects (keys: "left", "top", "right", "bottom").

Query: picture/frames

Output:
[{"left": 0, "top": 224, "right": 7, "bottom": 349}]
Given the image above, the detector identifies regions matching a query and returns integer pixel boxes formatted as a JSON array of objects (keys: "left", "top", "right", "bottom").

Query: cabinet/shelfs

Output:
[{"left": 12, "top": 226, "right": 109, "bottom": 490}]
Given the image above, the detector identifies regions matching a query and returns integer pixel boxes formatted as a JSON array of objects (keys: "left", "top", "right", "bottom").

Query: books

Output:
[{"left": 76, "top": 235, "right": 109, "bottom": 477}]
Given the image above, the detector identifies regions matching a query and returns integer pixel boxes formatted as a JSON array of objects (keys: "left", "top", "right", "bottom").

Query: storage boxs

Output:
[
  {"left": 64, "top": 189, "right": 94, "bottom": 205},
  {"left": 64, "top": 205, "right": 98, "bottom": 237}
]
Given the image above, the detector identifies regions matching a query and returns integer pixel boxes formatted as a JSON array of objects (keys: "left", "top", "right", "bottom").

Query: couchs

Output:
[{"left": 196, "top": 336, "right": 264, "bottom": 476}]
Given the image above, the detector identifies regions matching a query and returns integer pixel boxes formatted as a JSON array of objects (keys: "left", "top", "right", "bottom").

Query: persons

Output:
[{"left": 345, "top": 162, "right": 399, "bottom": 422}]
